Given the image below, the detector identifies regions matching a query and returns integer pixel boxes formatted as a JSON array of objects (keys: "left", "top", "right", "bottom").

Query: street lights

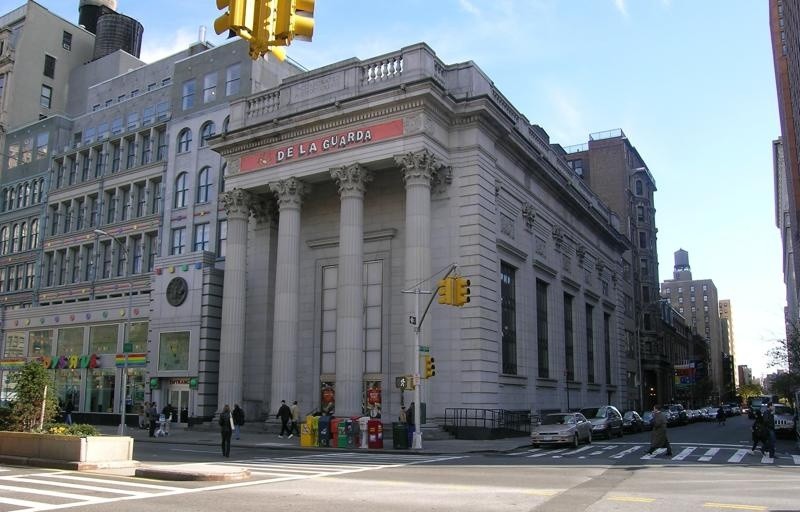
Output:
[{"left": 92, "top": 227, "right": 133, "bottom": 436}]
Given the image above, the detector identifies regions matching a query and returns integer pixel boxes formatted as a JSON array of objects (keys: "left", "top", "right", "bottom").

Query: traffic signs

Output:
[{"left": 419, "top": 345, "right": 430, "bottom": 352}]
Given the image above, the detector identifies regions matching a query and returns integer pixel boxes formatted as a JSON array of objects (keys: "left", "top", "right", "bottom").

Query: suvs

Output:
[{"left": 771, "top": 401, "right": 797, "bottom": 441}]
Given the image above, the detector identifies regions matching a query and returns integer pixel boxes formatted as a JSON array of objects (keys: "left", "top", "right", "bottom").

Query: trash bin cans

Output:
[{"left": 393, "top": 422, "right": 408, "bottom": 450}]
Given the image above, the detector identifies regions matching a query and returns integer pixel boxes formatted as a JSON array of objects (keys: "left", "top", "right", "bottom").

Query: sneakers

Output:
[
  {"left": 748, "top": 450, "right": 754, "bottom": 455},
  {"left": 769, "top": 456, "right": 778, "bottom": 458},
  {"left": 644, "top": 450, "right": 652, "bottom": 454},
  {"left": 665, "top": 453, "right": 672, "bottom": 456},
  {"left": 279, "top": 435, "right": 283, "bottom": 439},
  {"left": 760, "top": 449, "right": 765, "bottom": 454},
  {"left": 288, "top": 434, "right": 293, "bottom": 439},
  {"left": 236, "top": 438, "right": 239, "bottom": 440},
  {"left": 226, "top": 454, "right": 229, "bottom": 457},
  {"left": 223, "top": 454, "right": 225, "bottom": 457}
]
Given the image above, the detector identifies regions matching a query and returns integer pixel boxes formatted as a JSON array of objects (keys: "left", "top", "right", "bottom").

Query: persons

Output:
[
  {"left": 643, "top": 403, "right": 672, "bottom": 456},
  {"left": 716, "top": 404, "right": 726, "bottom": 426},
  {"left": 400, "top": 406, "right": 407, "bottom": 423},
  {"left": 65, "top": 399, "right": 75, "bottom": 426},
  {"left": 218, "top": 405, "right": 234, "bottom": 458},
  {"left": 407, "top": 402, "right": 415, "bottom": 426},
  {"left": 760, "top": 402, "right": 778, "bottom": 458},
  {"left": 368, "top": 403, "right": 382, "bottom": 418},
  {"left": 275, "top": 398, "right": 334, "bottom": 439},
  {"left": 126, "top": 395, "right": 197, "bottom": 439},
  {"left": 752, "top": 411, "right": 766, "bottom": 450},
  {"left": 231, "top": 404, "right": 243, "bottom": 440}
]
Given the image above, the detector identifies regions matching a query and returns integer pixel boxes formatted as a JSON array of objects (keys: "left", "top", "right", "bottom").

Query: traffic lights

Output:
[
  {"left": 451, "top": 276, "right": 471, "bottom": 308},
  {"left": 395, "top": 376, "right": 408, "bottom": 389},
  {"left": 437, "top": 276, "right": 452, "bottom": 305},
  {"left": 274, "top": 0, "right": 315, "bottom": 44},
  {"left": 212, "top": 0, "right": 259, "bottom": 38},
  {"left": 425, "top": 354, "right": 435, "bottom": 378}
]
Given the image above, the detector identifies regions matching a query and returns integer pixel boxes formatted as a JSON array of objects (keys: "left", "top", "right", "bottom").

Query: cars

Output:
[
  {"left": 619, "top": 407, "right": 645, "bottom": 436},
  {"left": 641, "top": 392, "right": 780, "bottom": 430},
  {"left": 528, "top": 411, "right": 594, "bottom": 449},
  {"left": 577, "top": 403, "right": 625, "bottom": 440}
]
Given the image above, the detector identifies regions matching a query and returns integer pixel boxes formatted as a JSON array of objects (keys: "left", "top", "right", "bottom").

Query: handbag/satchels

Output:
[{"left": 230, "top": 418, "right": 235, "bottom": 430}]
